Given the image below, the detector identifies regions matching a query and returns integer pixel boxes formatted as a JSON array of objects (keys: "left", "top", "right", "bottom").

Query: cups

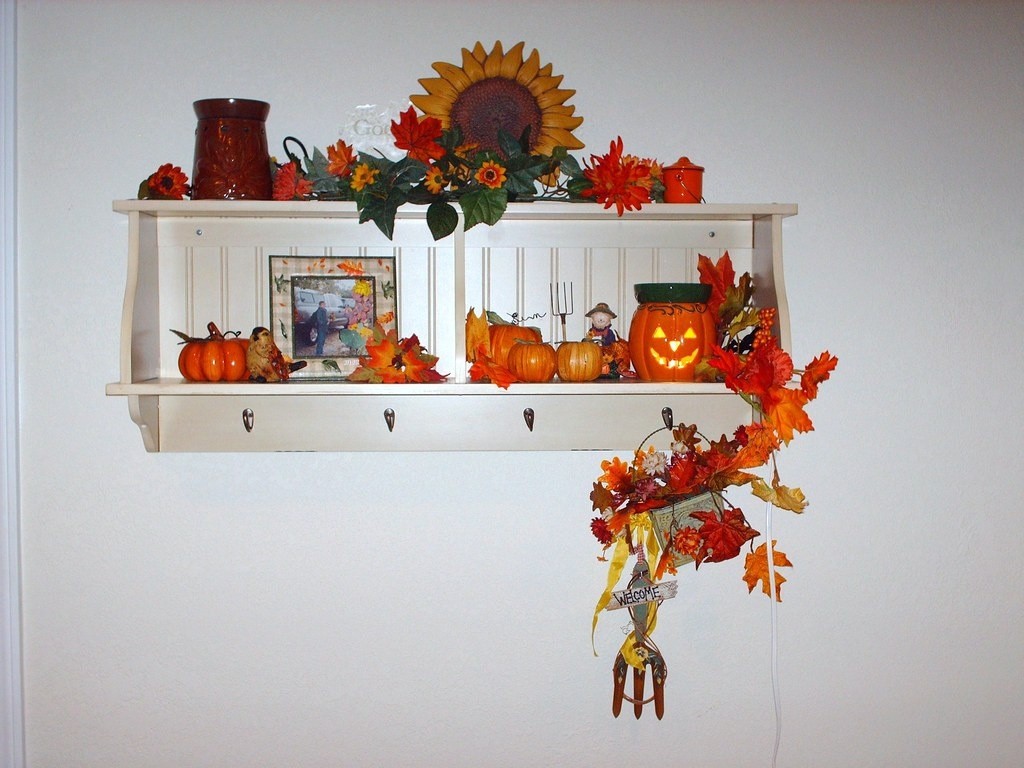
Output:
[{"left": 662, "top": 156, "right": 705, "bottom": 203}]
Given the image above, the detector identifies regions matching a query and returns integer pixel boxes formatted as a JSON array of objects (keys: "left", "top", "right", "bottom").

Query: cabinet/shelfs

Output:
[{"left": 106, "top": 200, "right": 799, "bottom": 453}]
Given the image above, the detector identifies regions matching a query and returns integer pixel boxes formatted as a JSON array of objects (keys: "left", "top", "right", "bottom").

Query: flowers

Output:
[
  {"left": 269, "top": 104, "right": 667, "bottom": 240},
  {"left": 139, "top": 164, "right": 191, "bottom": 200}
]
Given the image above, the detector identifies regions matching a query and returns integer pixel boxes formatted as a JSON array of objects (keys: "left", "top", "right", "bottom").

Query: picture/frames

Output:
[{"left": 269, "top": 255, "right": 398, "bottom": 377}]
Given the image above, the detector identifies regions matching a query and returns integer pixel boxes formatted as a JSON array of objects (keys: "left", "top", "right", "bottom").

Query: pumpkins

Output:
[
  {"left": 557, "top": 341, "right": 603, "bottom": 381},
  {"left": 508, "top": 339, "right": 556, "bottom": 381},
  {"left": 178, "top": 322, "right": 253, "bottom": 380},
  {"left": 487, "top": 325, "right": 539, "bottom": 367}
]
